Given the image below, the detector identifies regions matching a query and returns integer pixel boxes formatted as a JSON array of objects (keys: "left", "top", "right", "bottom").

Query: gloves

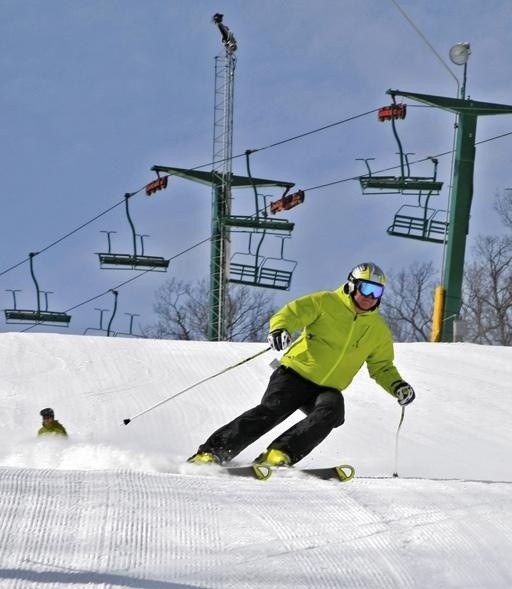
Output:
[
  {"left": 267, "top": 328, "right": 293, "bottom": 351},
  {"left": 394, "top": 382, "right": 416, "bottom": 406}
]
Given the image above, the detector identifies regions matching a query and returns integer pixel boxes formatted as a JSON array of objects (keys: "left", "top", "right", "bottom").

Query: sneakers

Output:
[
  {"left": 252, "top": 449, "right": 294, "bottom": 470},
  {"left": 186, "top": 450, "right": 222, "bottom": 467}
]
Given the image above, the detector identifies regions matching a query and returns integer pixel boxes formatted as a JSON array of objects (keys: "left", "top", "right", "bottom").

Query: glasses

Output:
[{"left": 347, "top": 274, "right": 384, "bottom": 300}]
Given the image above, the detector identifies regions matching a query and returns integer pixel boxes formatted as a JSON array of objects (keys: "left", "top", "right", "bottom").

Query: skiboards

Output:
[{"left": 223, "top": 465, "right": 355, "bottom": 482}]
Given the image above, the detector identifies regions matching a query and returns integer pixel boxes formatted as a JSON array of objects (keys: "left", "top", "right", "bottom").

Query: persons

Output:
[
  {"left": 186, "top": 262, "right": 416, "bottom": 468},
  {"left": 37, "top": 407, "right": 67, "bottom": 437}
]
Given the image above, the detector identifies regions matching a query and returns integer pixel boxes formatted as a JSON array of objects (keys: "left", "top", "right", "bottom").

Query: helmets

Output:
[
  {"left": 40, "top": 408, "right": 55, "bottom": 417},
  {"left": 344, "top": 262, "right": 387, "bottom": 295}
]
